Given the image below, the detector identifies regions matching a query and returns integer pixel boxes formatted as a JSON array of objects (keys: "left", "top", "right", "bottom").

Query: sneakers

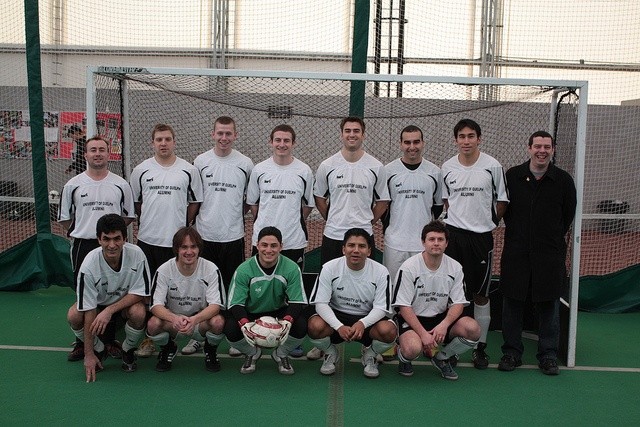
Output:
[
  {"left": 182, "top": 338, "right": 205, "bottom": 354},
  {"left": 229, "top": 346, "right": 243, "bottom": 356},
  {"left": 473, "top": 342, "right": 489, "bottom": 368},
  {"left": 382, "top": 343, "right": 398, "bottom": 361},
  {"left": 204, "top": 338, "right": 221, "bottom": 371},
  {"left": 138, "top": 339, "right": 155, "bottom": 357},
  {"left": 361, "top": 353, "right": 379, "bottom": 378},
  {"left": 271, "top": 348, "right": 294, "bottom": 375},
  {"left": 431, "top": 355, "right": 458, "bottom": 380},
  {"left": 94, "top": 344, "right": 109, "bottom": 372},
  {"left": 109, "top": 340, "right": 123, "bottom": 359},
  {"left": 121, "top": 348, "right": 137, "bottom": 371},
  {"left": 423, "top": 347, "right": 438, "bottom": 357},
  {"left": 361, "top": 344, "right": 384, "bottom": 363},
  {"left": 307, "top": 347, "right": 323, "bottom": 360},
  {"left": 240, "top": 346, "right": 263, "bottom": 373},
  {"left": 288, "top": 345, "right": 305, "bottom": 357},
  {"left": 157, "top": 336, "right": 179, "bottom": 371},
  {"left": 320, "top": 345, "right": 339, "bottom": 374},
  {"left": 68, "top": 342, "right": 83, "bottom": 361},
  {"left": 450, "top": 353, "right": 460, "bottom": 367},
  {"left": 399, "top": 359, "right": 414, "bottom": 376}
]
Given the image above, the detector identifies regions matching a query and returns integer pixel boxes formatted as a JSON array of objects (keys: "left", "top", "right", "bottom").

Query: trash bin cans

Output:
[
  {"left": 0, "top": 180, "right": 17, "bottom": 216},
  {"left": 597, "top": 200, "right": 630, "bottom": 234}
]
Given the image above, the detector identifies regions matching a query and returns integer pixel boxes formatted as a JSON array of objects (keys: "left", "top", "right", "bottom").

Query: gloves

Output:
[
  {"left": 276, "top": 315, "right": 294, "bottom": 345},
  {"left": 238, "top": 318, "right": 257, "bottom": 347}
]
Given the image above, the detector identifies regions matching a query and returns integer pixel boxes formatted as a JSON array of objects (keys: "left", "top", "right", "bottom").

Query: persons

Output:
[
  {"left": 67, "top": 213, "right": 152, "bottom": 384},
  {"left": 57, "top": 136, "right": 136, "bottom": 361},
  {"left": 380, "top": 124, "right": 444, "bottom": 360},
  {"left": 246, "top": 124, "right": 315, "bottom": 357},
  {"left": 145, "top": 226, "right": 227, "bottom": 372},
  {"left": 306, "top": 117, "right": 391, "bottom": 363},
  {"left": 497, "top": 130, "right": 577, "bottom": 375},
  {"left": 64, "top": 124, "right": 86, "bottom": 176},
  {"left": 130, "top": 124, "right": 204, "bottom": 357},
  {"left": 391, "top": 220, "right": 482, "bottom": 380},
  {"left": 225, "top": 225, "right": 308, "bottom": 375},
  {"left": 441, "top": 118, "right": 511, "bottom": 370},
  {"left": 181, "top": 116, "right": 255, "bottom": 356},
  {"left": 307, "top": 227, "right": 398, "bottom": 378}
]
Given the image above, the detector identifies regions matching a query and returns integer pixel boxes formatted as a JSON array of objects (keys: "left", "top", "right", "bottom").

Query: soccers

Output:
[
  {"left": 49, "top": 190, "right": 60, "bottom": 200},
  {"left": 251, "top": 316, "right": 286, "bottom": 350}
]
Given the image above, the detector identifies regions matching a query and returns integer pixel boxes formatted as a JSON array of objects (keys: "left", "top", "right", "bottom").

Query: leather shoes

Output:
[
  {"left": 539, "top": 360, "right": 559, "bottom": 374},
  {"left": 498, "top": 355, "right": 521, "bottom": 371}
]
